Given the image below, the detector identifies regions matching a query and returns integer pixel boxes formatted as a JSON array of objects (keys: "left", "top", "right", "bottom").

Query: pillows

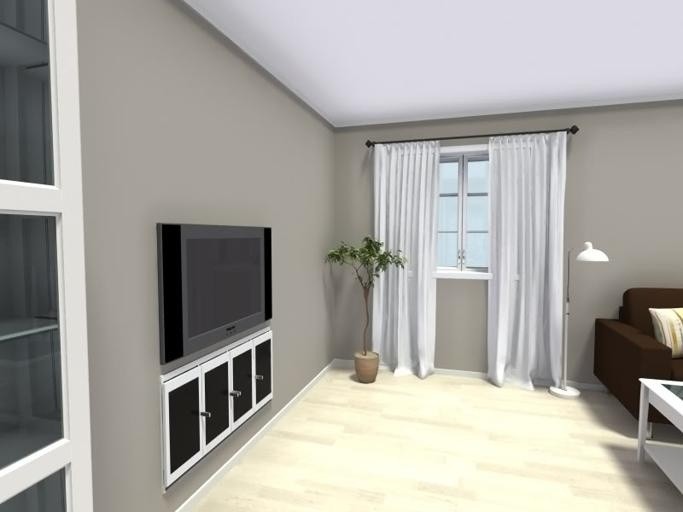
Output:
[{"left": 648, "top": 308, "right": 683, "bottom": 359}]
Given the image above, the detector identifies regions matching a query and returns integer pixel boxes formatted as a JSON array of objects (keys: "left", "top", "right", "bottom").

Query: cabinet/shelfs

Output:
[
  {"left": 0, "top": 0, "right": 94, "bottom": 512},
  {"left": 160, "top": 327, "right": 274, "bottom": 491}
]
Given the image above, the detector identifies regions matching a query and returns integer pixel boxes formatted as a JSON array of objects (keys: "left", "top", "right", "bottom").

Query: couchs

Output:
[{"left": 594, "top": 287, "right": 683, "bottom": 425}]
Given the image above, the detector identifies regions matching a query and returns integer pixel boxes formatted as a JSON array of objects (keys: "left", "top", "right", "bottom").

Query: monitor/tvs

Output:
[{"left": 180, "top": 224, "right": 266, "bottom": 357}]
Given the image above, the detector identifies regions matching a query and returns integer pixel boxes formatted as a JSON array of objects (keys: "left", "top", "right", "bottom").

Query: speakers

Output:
[
  {"left": 264, "top": 227, "right": 272, "bottom": 321},
  {"left": 157, "top": 223, "right": 184, "bottom": 365}
]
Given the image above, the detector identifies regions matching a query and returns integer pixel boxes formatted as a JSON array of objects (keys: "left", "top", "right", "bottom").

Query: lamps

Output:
[{"left": 548, "top": 241, "right": 609, "bottom": 400}]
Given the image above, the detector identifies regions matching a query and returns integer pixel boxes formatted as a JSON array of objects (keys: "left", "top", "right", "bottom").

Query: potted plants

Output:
[{"left": 325, "top": 235, "right": 407, "bottom": 383}]
